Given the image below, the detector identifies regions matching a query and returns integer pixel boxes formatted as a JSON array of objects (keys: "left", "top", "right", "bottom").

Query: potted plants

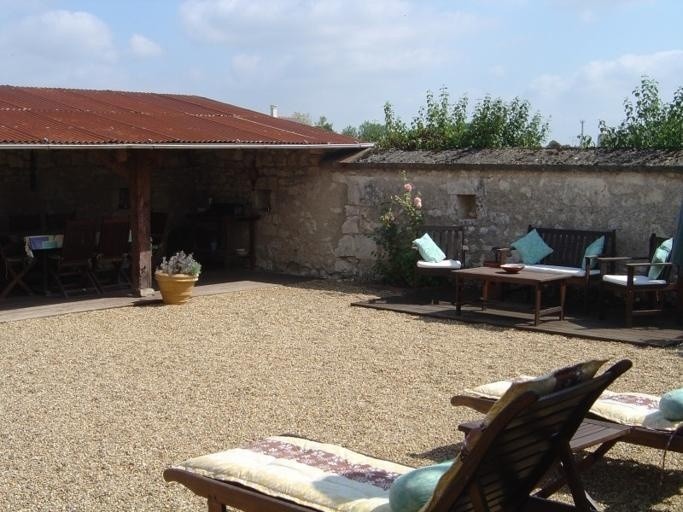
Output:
[{"left": 153, "top": 250, "right": 202, "bottom": 306}]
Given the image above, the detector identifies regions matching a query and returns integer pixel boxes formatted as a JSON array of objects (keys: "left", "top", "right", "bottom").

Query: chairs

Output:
[
  {"left": 2, "top": 236, "right": 131, "bottom": 303},
  {"left": 447, "top": 369, "right": 683, "bottom": 491},
  {"left": 596, "top": 232, "right": 675, "bottom": 328},
  {"left": 162, "top": 356, "right": 639, "bottom": 511},
  {"left": 412, "top": 224, "right": 465, "bottom": 308}
]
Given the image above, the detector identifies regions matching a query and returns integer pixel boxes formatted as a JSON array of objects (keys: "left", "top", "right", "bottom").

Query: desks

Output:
[
  {"left": 455, "top": 416, "right": 634, "bottom": 502},
  {"left": 449, "top": 264, "right": 572, "bottom": 326}
]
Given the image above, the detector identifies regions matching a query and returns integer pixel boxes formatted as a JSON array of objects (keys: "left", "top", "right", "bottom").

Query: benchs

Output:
[{"left": 491, "top": 224, "right": 616, "bottom": 323}]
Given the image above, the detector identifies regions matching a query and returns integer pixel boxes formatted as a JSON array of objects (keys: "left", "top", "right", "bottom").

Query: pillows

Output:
[
  {"left": 510, "top": 228, "right": 555, "bottom": 264},
  {"left": 386, "top": 452, "right": 464, "bottom": 511},
  {"left": 652, "top": 382, "right": 683, "bottom": 418},
  {"left": 578, "top": 234, "right": 607, "bottom": 270},
  {"left": 647, "top": 234, "right": 673, "bottom": 281},
  {"left": 412, "top": 230, "right": 445, "bottom": 261}
]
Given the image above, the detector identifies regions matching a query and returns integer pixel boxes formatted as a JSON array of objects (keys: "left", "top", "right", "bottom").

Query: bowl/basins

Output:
[{"left": 500, "top": 264, "right": 524, "bottom": 273}]
